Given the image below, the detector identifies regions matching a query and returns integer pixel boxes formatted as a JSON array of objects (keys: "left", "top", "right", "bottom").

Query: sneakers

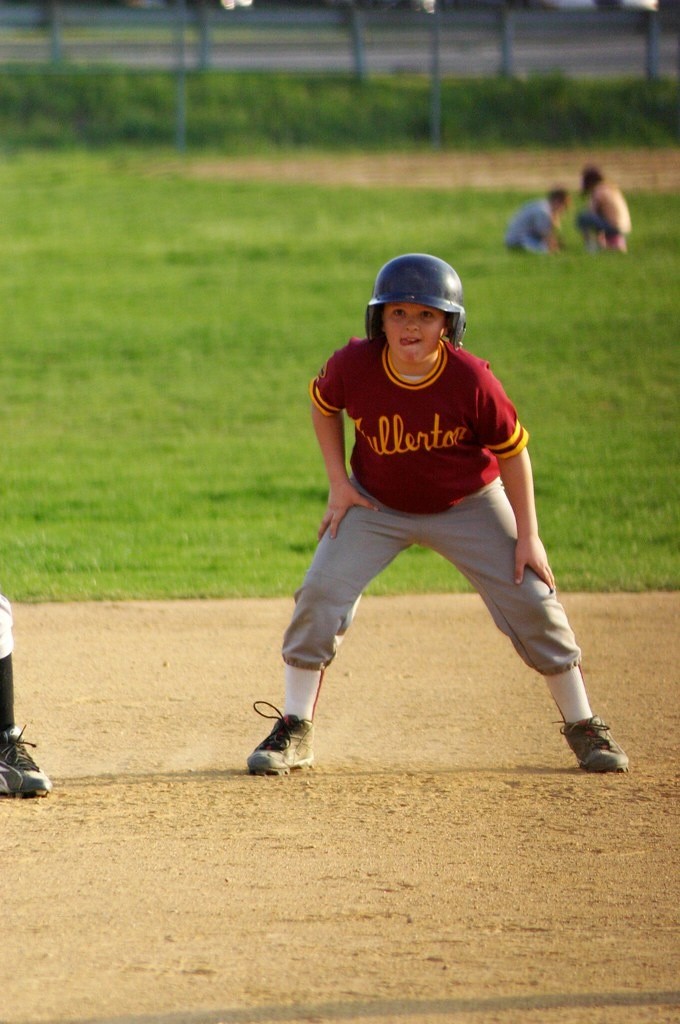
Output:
[
  {"left": 0, "top": 723, "right": 52, "bottom": 794},
  {"left": 560, "top": 714, "right": 629, "bottom": 772},
  {"left": 247, "top": 701, "right": 315, "bottom": 776}
]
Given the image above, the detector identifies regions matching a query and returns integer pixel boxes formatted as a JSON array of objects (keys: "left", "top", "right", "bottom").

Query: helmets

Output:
[{"left": 365, "top": 253, "right": 466, "bottom": 352}]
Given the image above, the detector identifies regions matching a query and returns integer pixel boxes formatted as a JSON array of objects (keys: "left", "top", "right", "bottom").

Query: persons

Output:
[
  {"left": 575, "top": 165, "right": 632, "bottom": 254},
  {"left": 0, "top": 595, "right": 51, "bottom": 798},
  {"left": 506, "top": 189, "right": 570, "bottom": 254},
  {"left": 248, "top": 254, "right": 629, "bottom": 776}
]
